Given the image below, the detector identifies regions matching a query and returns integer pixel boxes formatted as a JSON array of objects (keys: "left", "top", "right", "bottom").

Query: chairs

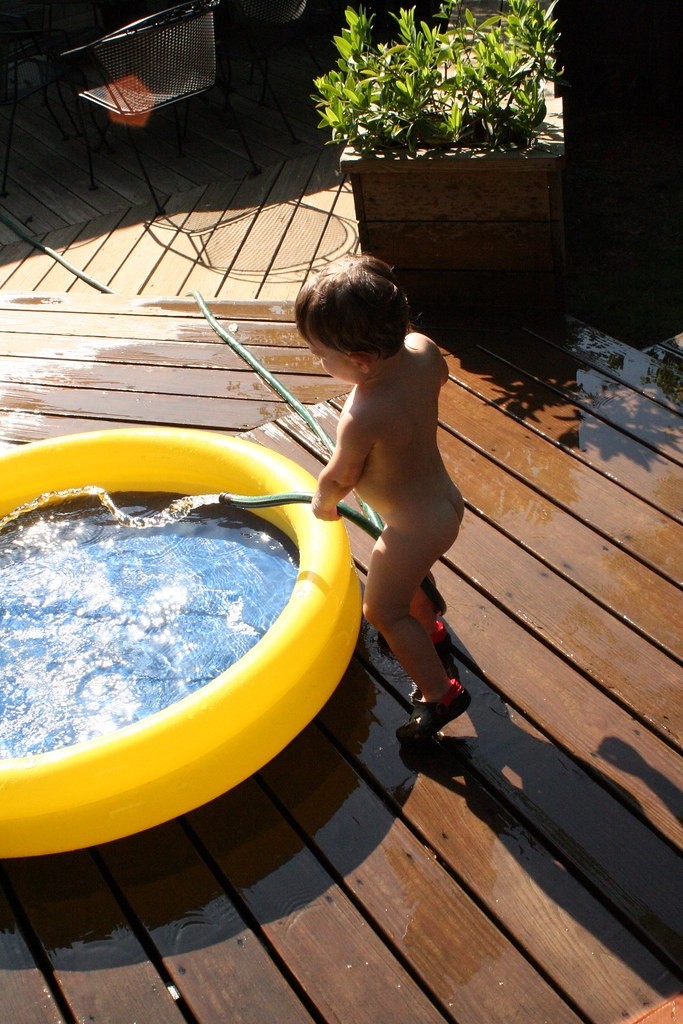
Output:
[
  {"left": 214, "top": 0, "right": 325, "bottom": 123},
  {"left": 58, "top": 0, "right": 263, "bottom": 217},
  {"left": 0, "top": 11, "right": 86, "bottom": 200}
]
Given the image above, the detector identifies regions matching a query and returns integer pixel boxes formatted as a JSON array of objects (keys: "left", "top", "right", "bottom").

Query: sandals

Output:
[
  {"left": 395, "top": 679, "right": 471, "bottom": 747},
  {"left": 379, "top": 621, "right": 451, "bottom": 654}
]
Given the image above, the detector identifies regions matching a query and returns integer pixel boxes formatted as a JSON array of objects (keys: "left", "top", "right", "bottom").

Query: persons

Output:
[{"left": 295, "top": 257, "right": 473, "bottom": 745}]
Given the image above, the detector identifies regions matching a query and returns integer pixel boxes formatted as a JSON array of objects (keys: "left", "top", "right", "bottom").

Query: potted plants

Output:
[{"left": 306, "top": 0, "right": 572, "bottom": 314}]
